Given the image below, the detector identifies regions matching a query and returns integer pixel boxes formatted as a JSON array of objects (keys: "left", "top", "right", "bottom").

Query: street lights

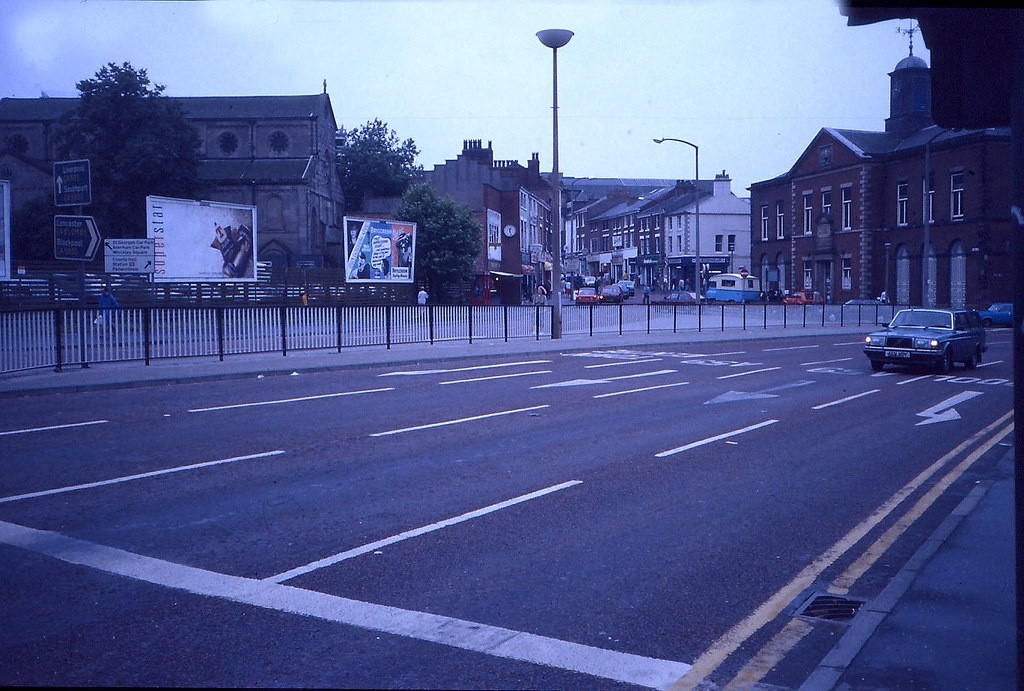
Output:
[
  {"left": 652, "top": 136, "right": 700, "bottom": 304},
  {"left": 536, "top": 29, "right": 576, "bottom": 338},
  {"left": 884, "top": 243, "right": 891, "bottom": 304},
  {"left": 571, "top": 176, "right": 594, "bottom": 299},
  {"left": 729, "top": 246, "right": 735, "bottom": 272},
  {"left": 921, "top": 126, "right": 963, "bottom": 309},
  {"left": 637, "top": 196, "right": 662, "bottom": 259}
]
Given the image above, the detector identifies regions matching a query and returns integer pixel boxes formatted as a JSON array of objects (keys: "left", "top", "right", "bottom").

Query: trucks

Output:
[{"left": 706, "top": 274, "right": 760, "bottom": 305}]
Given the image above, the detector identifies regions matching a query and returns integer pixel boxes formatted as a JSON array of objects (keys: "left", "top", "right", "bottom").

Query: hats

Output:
[{"left": 420, "top": 286, "right": 425, "bottom": 290}]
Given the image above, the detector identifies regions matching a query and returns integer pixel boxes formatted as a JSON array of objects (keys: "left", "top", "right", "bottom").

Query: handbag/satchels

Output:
[{"left": 94, "top": 315, "right": 103, "bottom": 325}]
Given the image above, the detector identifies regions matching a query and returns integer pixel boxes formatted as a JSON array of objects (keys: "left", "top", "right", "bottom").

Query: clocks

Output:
[{"left": 503, "top": 224, "right": 517, "bottom": 237}]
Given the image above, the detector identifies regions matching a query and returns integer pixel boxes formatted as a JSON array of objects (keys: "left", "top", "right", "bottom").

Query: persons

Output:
[
  {"left": 880, "top": 290, "right": 890, "bottom": 305},
  {"left": 99, "top": 287, "right": 120, "bottom": 337},
  {"left": 761, "top": 284, "right": 821, "bottom": 305},
  {"left": 300, "top": 293, "right": 307, "bottom": 306},
  {"left": 532, "top": 286, "right": 547, "bottom": 335},
  {"left": 560, "top": 270, "right": 628, "bottom": 298},
  {"left": 348, "top": 225, "right": 358, "bottom": 261},
  {"left": 640, "top": 278, "right": 691, "bottom": 304},
  {"left": 417, "top": 285, "right": 429, "bottom": 320}
]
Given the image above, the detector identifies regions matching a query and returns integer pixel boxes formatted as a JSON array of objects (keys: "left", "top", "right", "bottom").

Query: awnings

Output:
[
  {"left": 544, "top": 261, "right": 553, "bottom": 272},
  {"left": 522, "top": 265, "right": 535, "bottom": 275},
  {"left": 491, "top": 271, "right": 523, "bottom": 278}
]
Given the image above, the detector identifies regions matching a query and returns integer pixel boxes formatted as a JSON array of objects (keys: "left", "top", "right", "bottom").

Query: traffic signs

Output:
[
  {"left": 51, "top": 160, "right": 92, "bottom": 208},
  {"left": 52, "top": 214, "right": 101, "bottom": 261}
]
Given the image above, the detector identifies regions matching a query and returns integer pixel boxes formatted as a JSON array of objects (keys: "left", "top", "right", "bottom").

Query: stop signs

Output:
[{"left": 740, "top": 269, "right": 749, "bottom": 278}]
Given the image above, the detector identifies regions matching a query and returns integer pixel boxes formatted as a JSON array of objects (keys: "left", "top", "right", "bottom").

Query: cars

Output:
[
  {"left": 978, "top": 302, "right": 1014, "bottom": 327},
  {"left": 863, "top": 307, "right": 989, "bottom": 375},
  {"left": 561, "top": 272, "right": 636, "bottom": 305},
  {"left": 782, "top": 291, "right": 824, "bottom": 306},
  {"left": 820, "top": 298, "right": 885, "bottom": 326},
  {"left": 650, "top": 291, "right": 706, "bottom": 313}
]
